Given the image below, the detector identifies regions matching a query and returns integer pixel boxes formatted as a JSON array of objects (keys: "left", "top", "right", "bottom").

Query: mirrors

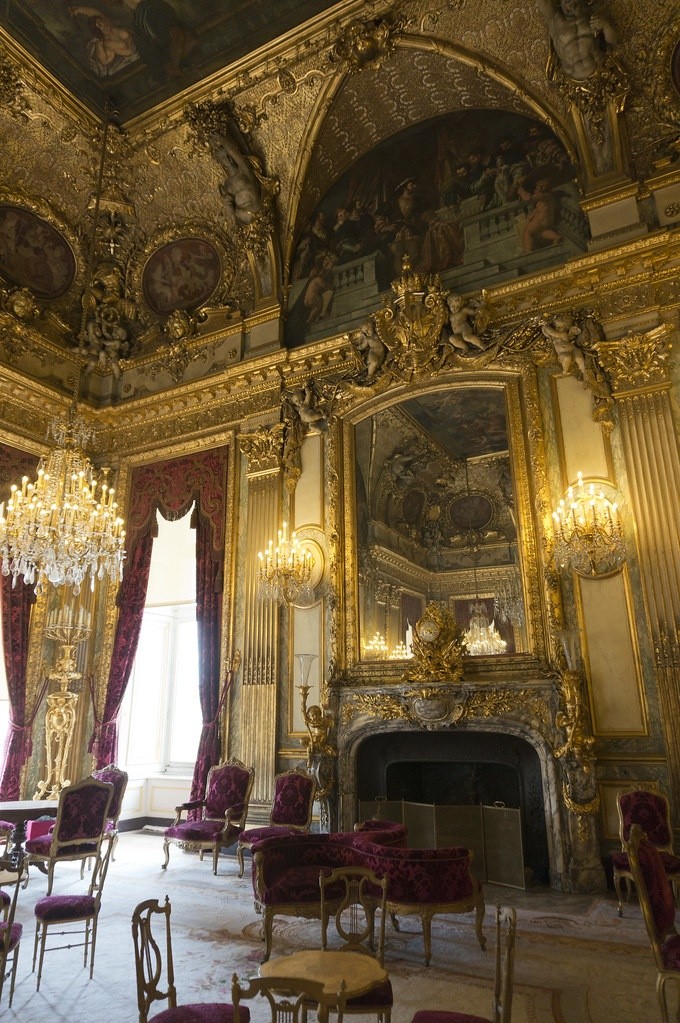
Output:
[{"left": 323, "top": 250, "right": 568, "bottom": 689}]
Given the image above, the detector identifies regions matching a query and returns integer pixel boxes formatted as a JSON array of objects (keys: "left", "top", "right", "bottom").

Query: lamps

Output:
[
  {"left": 31, "top": 602, "right": 94, "bottom": 802},
  {"left": 387, "top": 640, "right": 415, "bottom": 661},
  {"left": 362, "top": 631, "right": 388, "bottom": 660},
  {"left": 491, "top": 545, "right": 527, "bottom": 630},
  {"left": 461, "top": 500, "right": 508, "bottom": 657},
  {"left": 550, "top": 470, "right": 629, "bottom": 580},
  {"left": 1, "top": 76, "right": 127, "bottom": 597},
  {"left": 255, "top": 520, "right": 326, "bottom": 610}
]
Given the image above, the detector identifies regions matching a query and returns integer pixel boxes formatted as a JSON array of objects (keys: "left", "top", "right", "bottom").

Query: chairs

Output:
[
  {"left": 230, "top": 971, "right": 349, "bottom": 1023},
  {"left": 236, "top": 765, "right": 318, "bottom": 879},
  {"left": 22, "top": 775, "right": 116, "bottom": 897},
  {"left": 611, "top": 780, "right": 680, "bottom": 919},
  {"left": 625, "top": 823, "right": 680, "bottom": 1023},
  {"left": 86, "top": 762, "right": 129, "bottom": 871},
  {"left": 0, "top": 820, "right": 16, "bottom": 855},
  {"left": 33, "top": 827, "right": 118, "bottom": 993},
  {"left": 410, "top": 902, "right": 518, "bottom": 1023},
  {"left": 0, "top": 889, "right": 11, "bottom": 923},
  {"left": 0, "top": 852, "right": 23, "bottom": 1009},
  {"left": 161, "top": 754, "right": 255, "bottom": 876},
  {"left": 297, "top": 865, "right": 395, "bottom": 1023},
  {"left": 131, "top": 895, "right": 251, "bottom": 1023},
  {"left": 251, "top": 816, "right": 488, "bottom": 968}
]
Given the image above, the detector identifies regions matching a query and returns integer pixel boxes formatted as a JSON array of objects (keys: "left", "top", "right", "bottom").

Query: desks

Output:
[
  {"left": 257, "top": 948, "right": 389, "bottom": 1023},
  {"left": 0, "top": 799, "right": 60, "bottom": 890}
]
